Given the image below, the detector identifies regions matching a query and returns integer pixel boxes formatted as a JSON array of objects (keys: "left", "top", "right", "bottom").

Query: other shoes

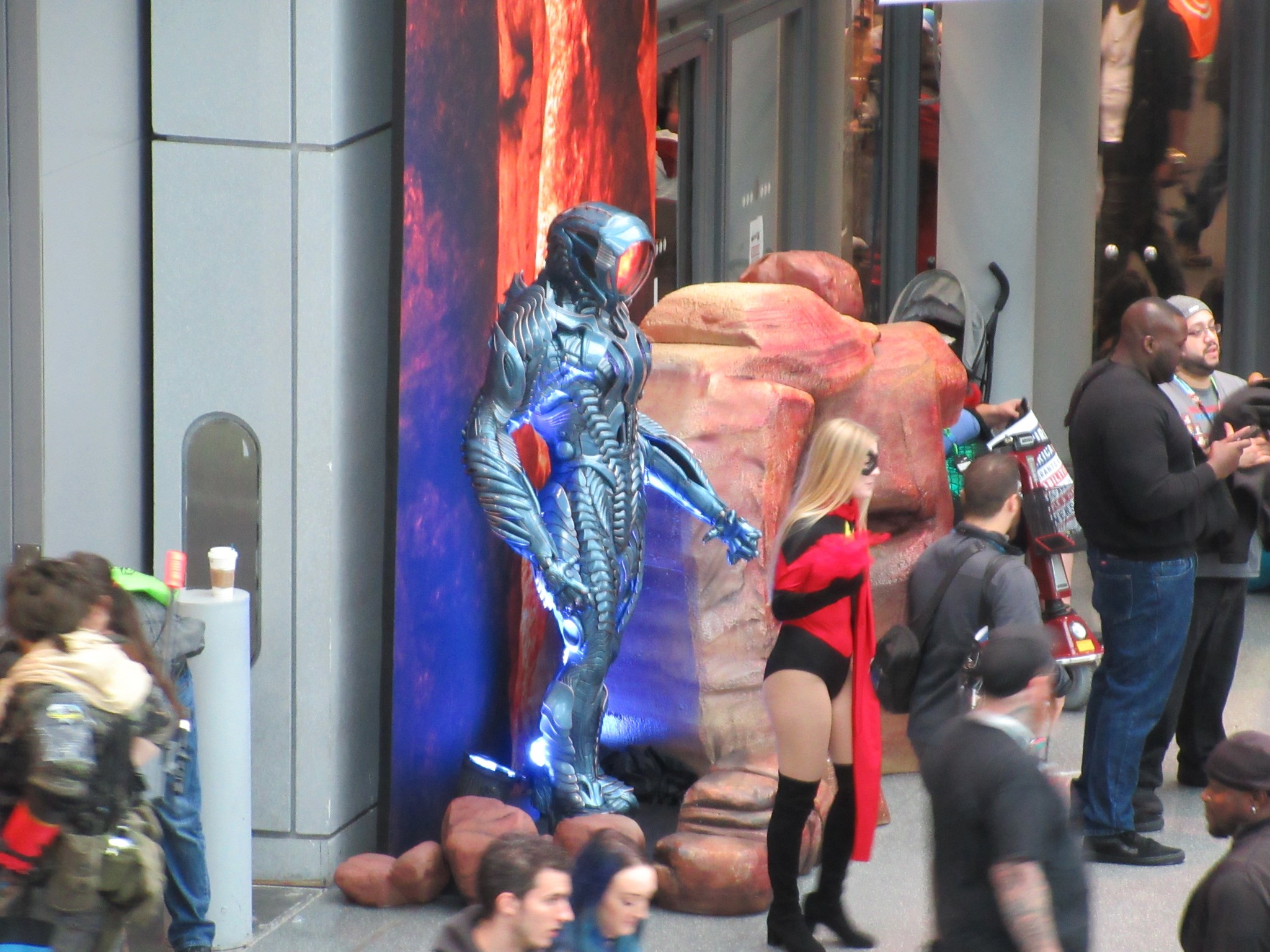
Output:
[
  {"left": 1177, "top": 236, "right": 1215, "bottom": 267},
  {"left": 1081, "top": 828, "right": 1185, "bottom": 866},
  {"left": 1178, "top": 768, "right": 1207, "bottom": 788},
  {"left": 1129, "top": 811, "right": 1167, "bottom": 832},
  {"left": 1134, "top": 789, "right": 1165, "bottom": 814}
]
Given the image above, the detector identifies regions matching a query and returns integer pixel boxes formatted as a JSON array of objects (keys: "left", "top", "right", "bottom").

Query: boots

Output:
[
  {"left": 765, "top": 768, "right": 827, "bottom": 952},
  {"left": 802, "top": 762, "right": 875, "bottom": 950}
]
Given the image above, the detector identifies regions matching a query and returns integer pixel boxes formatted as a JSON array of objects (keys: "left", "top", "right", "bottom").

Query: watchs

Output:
[{"left": 1163, "top": 150, "right": 1189, "bottom": 170}]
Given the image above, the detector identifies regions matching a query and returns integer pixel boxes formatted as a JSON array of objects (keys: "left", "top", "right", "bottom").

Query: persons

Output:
[
  {"left": 904, "top": 453, "right": 1049, "bottom": 796},
  {"left": 433, "top": 826, "right": 658, "bottom": 952},
  {"left": 943, "top": 398, "right": 1027, "bottom": 452},
  {"left": 1177, "top": 732, "right": 1270, "bottom": 952},
  {"left": 465, "top": 201, "right": 768, "bottom": 820},
  {"left": 1088, "top": 0, "right": 1268, "bottom": 871},
  {"left": 762, "top": 418, "right": 892, "bottom": 952},
  {"left": 920, "top": 623, "right": 1087, "bottom": 952},
  {"left": 64, "top": 550, "right": 215, "bottom": 952},
  {"left": 0, "top": 558, "right": 173, "bottom": 952}
]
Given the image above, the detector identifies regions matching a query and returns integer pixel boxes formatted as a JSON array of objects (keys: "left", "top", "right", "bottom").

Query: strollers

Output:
[{"left": 886, "top": 255, "right": 1011, "bottom": 405}]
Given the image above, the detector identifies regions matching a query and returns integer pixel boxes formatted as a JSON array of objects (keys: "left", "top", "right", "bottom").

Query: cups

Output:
[{"left": 208, "top": 545, "right": 239, "bottom": 599}]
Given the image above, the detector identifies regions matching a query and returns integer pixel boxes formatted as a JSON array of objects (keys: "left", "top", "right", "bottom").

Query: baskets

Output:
[{"left": 1022, "top": 483, "right": 1089, "bottom": 556}]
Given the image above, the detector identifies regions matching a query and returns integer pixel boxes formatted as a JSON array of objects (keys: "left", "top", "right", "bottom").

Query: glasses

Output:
[
  {"left": 861, "top": 452, "right": 879, "bottom": 476},
  {"left": 1187, "top": 323, "right": 1221, "bottom": 337}
]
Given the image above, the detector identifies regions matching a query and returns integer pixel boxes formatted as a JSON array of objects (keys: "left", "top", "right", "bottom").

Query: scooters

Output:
[{"left": 948, "top": 397, "right": 1104, "bottom": 712}]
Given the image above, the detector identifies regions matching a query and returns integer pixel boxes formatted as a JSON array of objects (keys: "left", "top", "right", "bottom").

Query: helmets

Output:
[{"left": 544, "top": 200, "right": 658, "bottom": 307}]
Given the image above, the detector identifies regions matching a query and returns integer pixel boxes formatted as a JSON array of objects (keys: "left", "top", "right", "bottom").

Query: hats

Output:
[
  {"left": 1165, "top": 294, "right": 1214, "bottom": 322},
  {"left": 1203, "top": 727, "right": 1270, "bottom": 792}
]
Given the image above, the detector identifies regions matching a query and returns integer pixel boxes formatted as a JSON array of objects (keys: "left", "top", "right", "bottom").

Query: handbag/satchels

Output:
[{"left": 867, "top": 622, "right": 921, "bottom": 715}]
